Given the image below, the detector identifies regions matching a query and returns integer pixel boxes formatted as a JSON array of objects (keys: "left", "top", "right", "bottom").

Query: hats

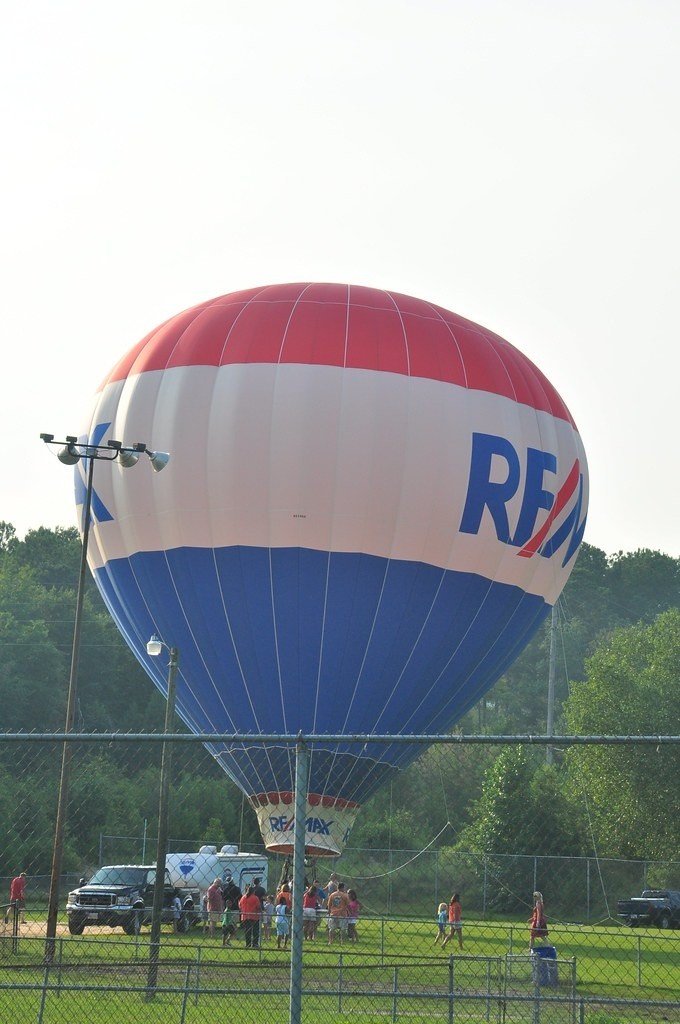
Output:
[{"left": 226, "top": 876, "right": 233, "bottom": 884}]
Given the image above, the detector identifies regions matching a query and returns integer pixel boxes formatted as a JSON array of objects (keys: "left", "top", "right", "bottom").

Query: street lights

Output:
[{"left": 41, "top": 432, "right": 174, "bottom": 969}]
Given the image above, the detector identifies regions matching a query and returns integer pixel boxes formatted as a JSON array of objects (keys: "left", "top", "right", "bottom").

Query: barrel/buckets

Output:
[{"left": 531, "top": 945, "right": 559, "bottom": 987}]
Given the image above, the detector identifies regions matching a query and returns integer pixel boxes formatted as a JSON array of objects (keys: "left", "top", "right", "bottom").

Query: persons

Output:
[
  {"left": 5, "top": 872, "right": 27, "bottom": 924},
  {"left": 526, "top": 891, "right": 556, "bottom": 952},
  {"left": 433, "top": 893, "right": 466, "bottom": 952},
  {"left": 172, "top": 867, "right": 359, "bottom": 949}
]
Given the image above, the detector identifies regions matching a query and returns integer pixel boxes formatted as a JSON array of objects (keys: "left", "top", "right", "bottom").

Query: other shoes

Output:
[
  {"left": 5, "top": 917, "right": 8, "bottom": 924},
  {"left": 252, "top": 944, "right": 262, "bottom": 948},
  {"left": 22, "top": 921, "right": 27, "bottom": 924},
  {"left": 225, "top": 940, "right": 231, "bottom": 946}
]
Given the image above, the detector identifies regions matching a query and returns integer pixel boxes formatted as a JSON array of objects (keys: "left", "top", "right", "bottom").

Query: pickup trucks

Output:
[
  {"left": 66, "top": 862, "right": 200, "bottom": 934},
  {"left": 618, "top": 890, "right": 680, "bottom": 928}
]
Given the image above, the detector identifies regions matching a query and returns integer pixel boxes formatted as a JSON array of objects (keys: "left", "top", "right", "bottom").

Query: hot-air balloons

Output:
[{"left": 75, "top": 285, "right": 589, "bottom": 932}]
